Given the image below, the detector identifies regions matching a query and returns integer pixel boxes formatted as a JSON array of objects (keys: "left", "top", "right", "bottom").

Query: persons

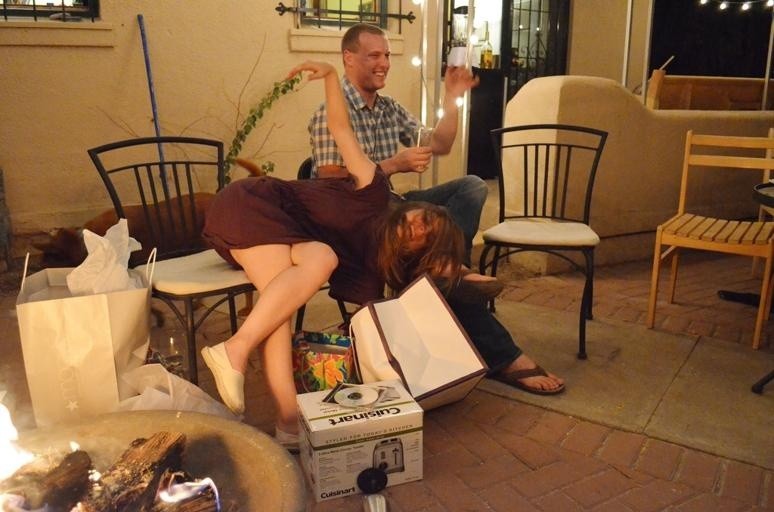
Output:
[
  {"left": 308, "top": 23, "right": 565, "bottom": 394},
  {"left": 201, "top": 60, "right": 466, "bottom": 452}
]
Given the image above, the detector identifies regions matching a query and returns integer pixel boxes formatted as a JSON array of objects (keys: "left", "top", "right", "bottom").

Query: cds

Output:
[{"left": 333, "top": 386, "right": 379, "bottom": 405}]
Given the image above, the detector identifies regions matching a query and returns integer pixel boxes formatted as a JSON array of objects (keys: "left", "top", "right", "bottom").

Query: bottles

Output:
[{"left": 480, "top": 31, "right": 494, "bottom": 69}]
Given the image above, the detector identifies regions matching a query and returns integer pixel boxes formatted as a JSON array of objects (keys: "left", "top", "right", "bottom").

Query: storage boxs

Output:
[{"left": 294, "top": 379, "right": 425, "bottom": 504}]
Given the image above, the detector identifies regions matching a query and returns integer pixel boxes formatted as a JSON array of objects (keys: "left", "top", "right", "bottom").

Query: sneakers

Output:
[
  {"left": 276, "top": 424, "right": 299, "bottom": 450},
  {"left": 201, "top": 342, "right": 245, "bottom": 417}
]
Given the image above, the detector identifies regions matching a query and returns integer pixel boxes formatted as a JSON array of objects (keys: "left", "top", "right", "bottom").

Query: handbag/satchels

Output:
[
  {"left": 349, "top": 272, "right": 490, "bottom": 412},
  {"left": 15, "top": 246, "right": 157, "bottom": 428},
  {"left": 292, "top": 330, "right": 356, "bottom": 394}
]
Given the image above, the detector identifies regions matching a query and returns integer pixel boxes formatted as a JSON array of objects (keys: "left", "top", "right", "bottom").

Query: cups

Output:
[
  {"left": 156, "top": 328, "right": 186, "bottom": 374},
  {"left": 411, "top": 126, "right": 433, "bottom": 168}
]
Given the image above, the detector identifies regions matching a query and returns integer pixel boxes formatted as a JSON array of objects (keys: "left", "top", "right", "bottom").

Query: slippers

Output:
[
  {"left": 433, "top": 268, "right": 504, "bottom": 304},
  {"left": 486, "top": 365, "right": 565, "bottom": 395}
]
